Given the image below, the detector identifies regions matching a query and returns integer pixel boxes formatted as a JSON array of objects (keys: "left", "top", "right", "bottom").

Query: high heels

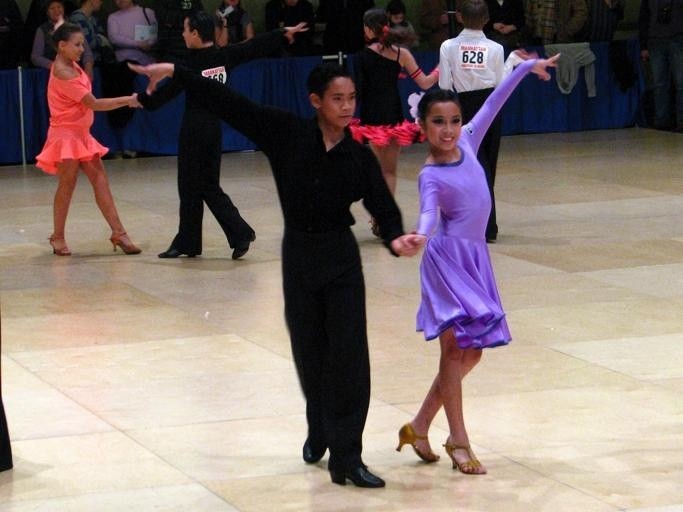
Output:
[
  {"left": 396, "top": 424, "right": 441, "bottom": 462},
  {"left": 49, "top": 235, "right": 70, "bottom": 255},
  {"left": 444, "top": 438, "right": 486, "bottom": 474},
  {"left": 110, "top": 233, "right": 141, "bottom": 255}
]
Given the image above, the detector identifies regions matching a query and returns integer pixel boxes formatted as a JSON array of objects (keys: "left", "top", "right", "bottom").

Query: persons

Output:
[
  {"left": 129, "top": 11, "right": 308, "bottom": 259},
  {"left": 126, "top": 61, "right": 412, "bottom": 489},
  {"left": 107, "top": 0, "right": 158, "bottom": 64},
  {"left": 395, "top": 53, "right": 563, "bottom": 474},
  {"left": 415, "top": 1, "right": 465, "bottom": 53},
  {"left": 439, "top": 1, "right": 537, "bottom": 245},
  {"left": 30, "top": 1, "right": 94, "bottom": 83},
  {"left": 266, "top": 0, "right": 320, "bottom": 60},
  {"left": 638, "top": 0, "right": 683, "bottom": 133},
  {"left": 380, "top": 3, "right": 418, "bottom": 52},
  {"left": 37, "top": 15, "right": 142, "bottom": 256},
  {"left": 454, "top": 1, "right": 525, "bottom": 51},
  {"left": 345, "top": 9, "right": 441, "bottom": 236},
  {"left": 213, "top": 0, "right": 256, "bottom": 62},
  {"left": 525, "top": 1, "right": 588, "bottom": 47},
  {"left": 69, "top": 0, "right": 112, "bottom": 66},
  {"left": 316, "top": 2, "right": 378, "bottom": 61}
]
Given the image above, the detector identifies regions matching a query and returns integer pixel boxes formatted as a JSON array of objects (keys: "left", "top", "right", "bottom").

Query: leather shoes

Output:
[
  {"left": 303, "top": 436, "right": 325, "bottom": 463},
  {"left": 330, "top": 463, "right": 385, "bottom": 488},
  {"left": 232, "top": 232, "right": 256, "bottom": 259},
  {"left": 159, "top": 245, "right": 196, "bottom": 257}
]
